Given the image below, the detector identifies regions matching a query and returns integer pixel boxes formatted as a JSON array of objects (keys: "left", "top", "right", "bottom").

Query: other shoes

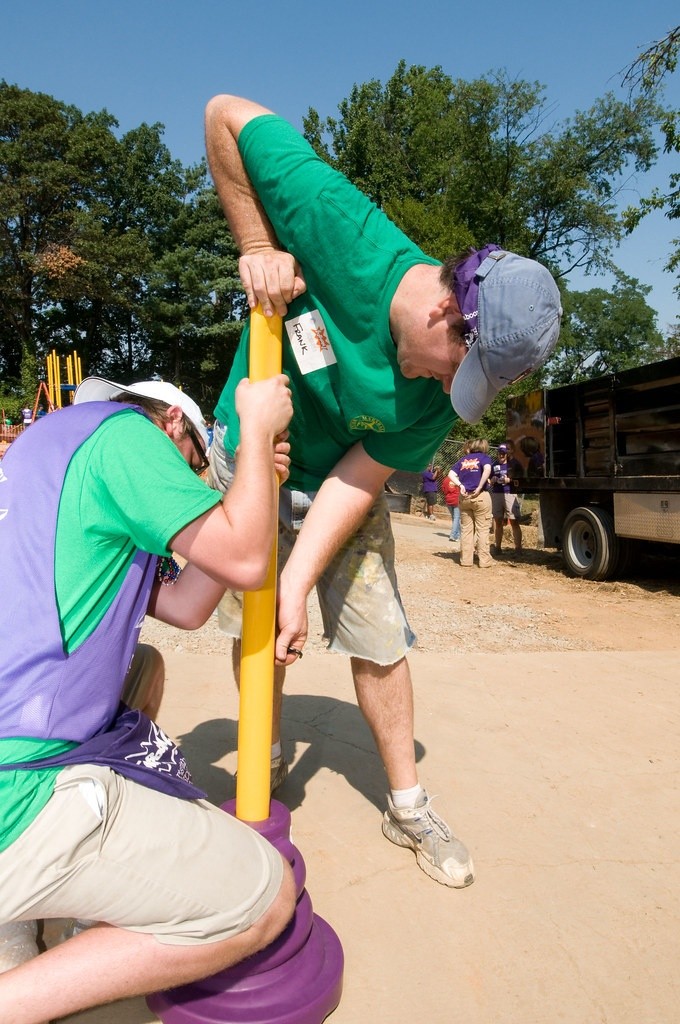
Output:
[{"left": 450, "top": 538, "right": 458, "bottom": 542}]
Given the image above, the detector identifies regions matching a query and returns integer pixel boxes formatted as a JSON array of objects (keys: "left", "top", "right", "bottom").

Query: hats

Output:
[
  {"left": 72, "top": 376, "right": 209, "bottom": 451},
  {"left": 450, "top": 250, "right": 563, "bottom": 425},
  {"left": 498, "top": 444, "right": 507, "bottom": 453}
]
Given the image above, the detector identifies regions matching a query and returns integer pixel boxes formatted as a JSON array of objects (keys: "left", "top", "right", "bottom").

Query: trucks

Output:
[{"left": 505, "top": 356, "right": 680, "bottom": 579}]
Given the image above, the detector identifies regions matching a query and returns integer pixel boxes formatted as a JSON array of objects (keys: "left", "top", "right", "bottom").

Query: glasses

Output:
[{"left": 186, "top": 426, "right": 211, "bottom": 476}]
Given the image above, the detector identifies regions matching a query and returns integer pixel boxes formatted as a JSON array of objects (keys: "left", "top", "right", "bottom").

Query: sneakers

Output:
[
  {"left": 381, "top": 792, "right": 475, "bottom": 889},
  {"left": 233, "top": 753, "right": 289, "bottom": 793}
]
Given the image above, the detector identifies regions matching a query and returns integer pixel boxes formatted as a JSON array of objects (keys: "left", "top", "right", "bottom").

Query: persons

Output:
[
  {"left": 489, "top": 443, "right": 522, "bottom": 559},
  {"left": 448, "top": 437, "right": 498, "bottom": 566},
  {"left": 5, "top": 403, "right": 47, "bottom": 430},
  {"left": 0, "top": 376, "right": 298, "bottom": 1023},
  {"left": 202, "top": 93, "right": 566, "bottom": 889},
  {"left": 204, "top": 421, "right": 214, "bottom": 464},
  {"left": 422, "top": 463, "right": 440, "bottom": 522},
  {"left": 441, "top": 473, "right": 462, "bottom": 542}
]
